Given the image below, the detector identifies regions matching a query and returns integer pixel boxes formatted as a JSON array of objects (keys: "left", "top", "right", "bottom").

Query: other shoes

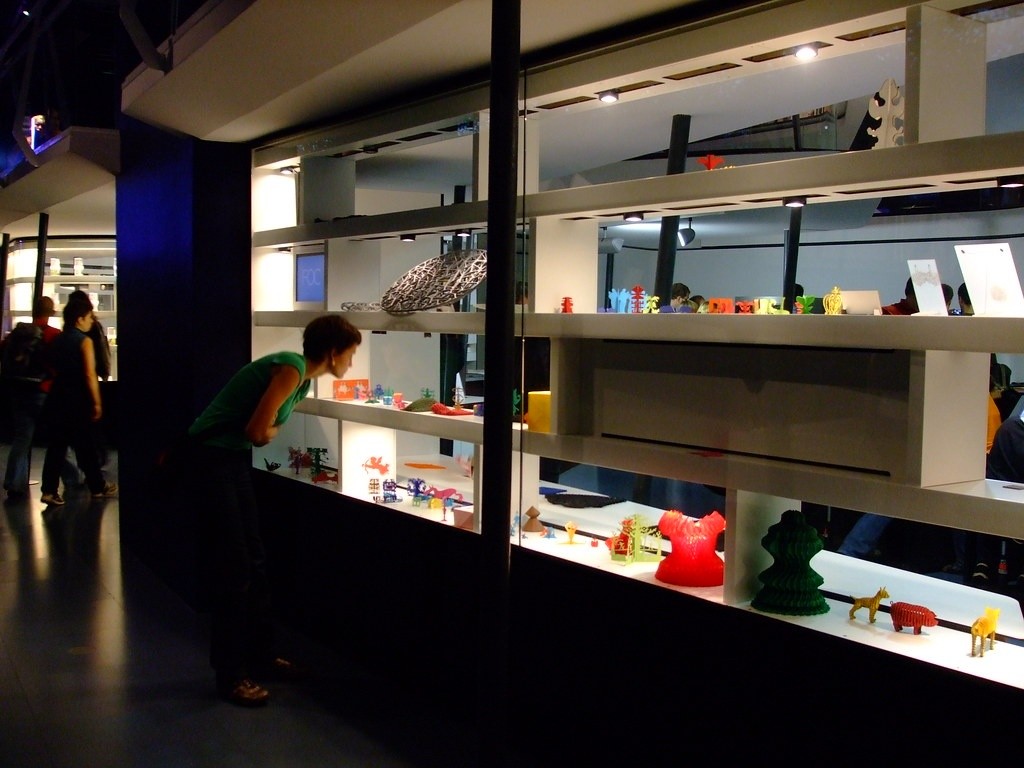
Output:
[
  {"left": 41, "top": 489, "right": 66, "bottom": 506},
  {"left": 250, "top": 656, "right": 310, "bottom": 681},
  {"left": 63, "top": 485, "right": 87, "bottom": 501},
  {"left": 83, "top": 471, "right": 109, "bottom": 490},
  {"left": 7, "top": 489, "right": 27, "bottom": 505},
  {"left": 216, "top": 678, "right": 272, "bottom": 706},
  {"left": 91, "top": 481, "right": 118, "bottom": 498}
]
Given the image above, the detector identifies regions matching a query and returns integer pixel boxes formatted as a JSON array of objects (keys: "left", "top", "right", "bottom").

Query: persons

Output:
[
  {"left": 985, "top": 354, "right": 1024, "bottom": 484},
  {"left": 185, "top": 314, "right": 363, "bottom": 709},
  {"left": 882, "top": 277, "right": 919, "bottom": 315},
  {"left": 41, "top": 291, "right": 119, "bottom": 507},
  {"left": 941, "top": 283, "right": 973, "bottom": 317},
  {"left": 793, "top": 284, "right": 804, "bottom": 312},
  {"left": 838, "top": 511, "right": 1024, "bottom": 591},
  {"left": 0, "top": 296, "right": 82, "bottom": 498},
  {"left": 669, "top": 281, "right": 705, "bottom": 313}
]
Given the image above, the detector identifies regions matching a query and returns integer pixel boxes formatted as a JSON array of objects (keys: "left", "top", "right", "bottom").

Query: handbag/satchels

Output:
[{"left": 165, "top": 426, "right": 195, "bottom": 476}]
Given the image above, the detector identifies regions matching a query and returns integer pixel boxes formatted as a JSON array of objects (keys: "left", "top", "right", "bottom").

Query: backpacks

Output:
[{"left": 4, "top": 323, "right": 48, "bottom": 376}]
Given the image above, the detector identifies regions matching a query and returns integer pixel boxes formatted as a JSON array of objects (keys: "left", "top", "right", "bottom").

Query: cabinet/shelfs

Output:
[{"left": 245, "top": 0, "right": 1024, "bottom": 693}]
